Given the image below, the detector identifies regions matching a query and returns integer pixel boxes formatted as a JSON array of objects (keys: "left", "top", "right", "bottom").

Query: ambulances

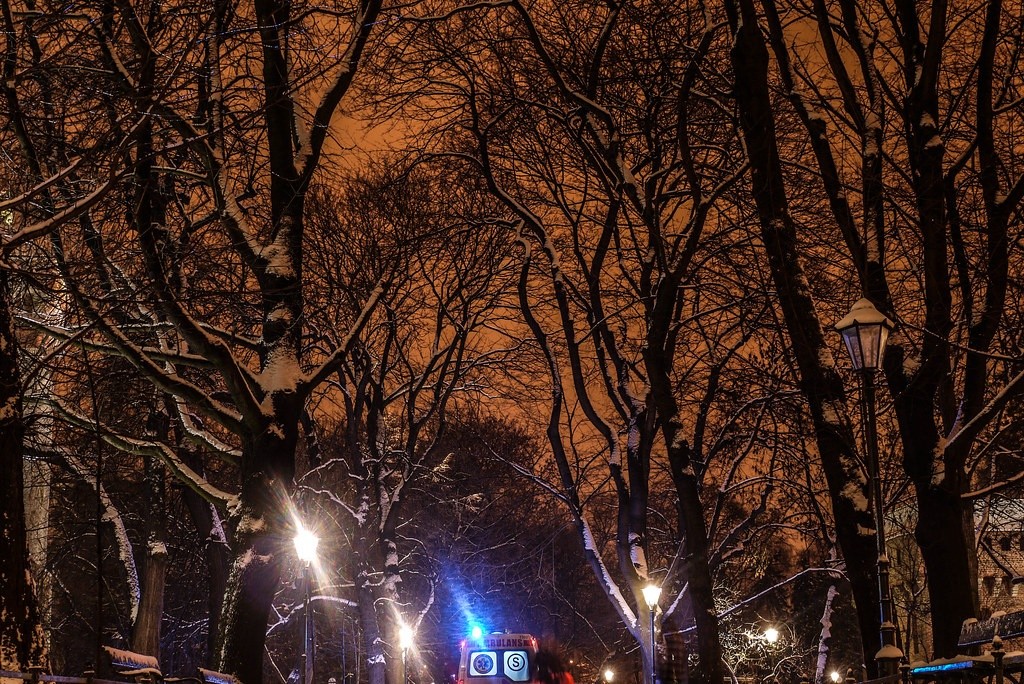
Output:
[{"left": 452, "top": 628, "right": 543, "bottom": 683}]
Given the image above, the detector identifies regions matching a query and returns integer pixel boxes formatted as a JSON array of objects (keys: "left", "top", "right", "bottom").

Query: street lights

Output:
[
  {"left": 601, "top": 665, "right": 617, "bottom": 681},
  {"left": 638, "top": 574, "right": 661, "bottom": 680},
  {"left": 291, "top": 519, "right": 319, "bottom": 680},
  {"left": 393, "top": 624, "right": 416, "bottom": 682},
  {"left": 831, "top": 294, "right": 905, "bottom": 683}
]
